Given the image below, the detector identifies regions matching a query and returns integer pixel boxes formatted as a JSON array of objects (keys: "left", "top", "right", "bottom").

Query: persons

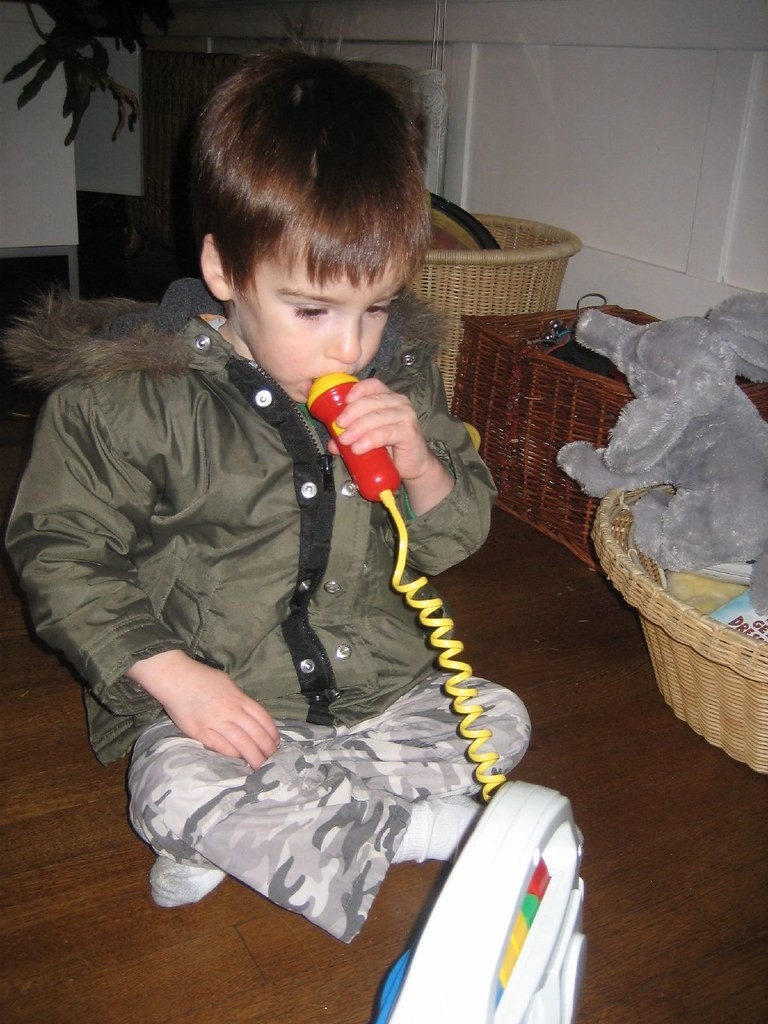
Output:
[{"left": 5, "top": 33, "right": 532, "bottom": 944}]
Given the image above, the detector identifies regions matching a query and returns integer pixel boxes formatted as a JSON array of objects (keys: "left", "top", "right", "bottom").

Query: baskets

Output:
[
  {"left": 591, "top": 479, "right": 768, "bottom": 772},
  {"left": 402, "top": 192, "right": 581, "bottom": 415},
  {"left": 449, "top": 292, "right": 768, "bottom": 576}
]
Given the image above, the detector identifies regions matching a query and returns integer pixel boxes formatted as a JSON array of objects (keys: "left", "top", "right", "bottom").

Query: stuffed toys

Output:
[{"left": 557, "top": 292, "right": 768, "bottom": 613}]
